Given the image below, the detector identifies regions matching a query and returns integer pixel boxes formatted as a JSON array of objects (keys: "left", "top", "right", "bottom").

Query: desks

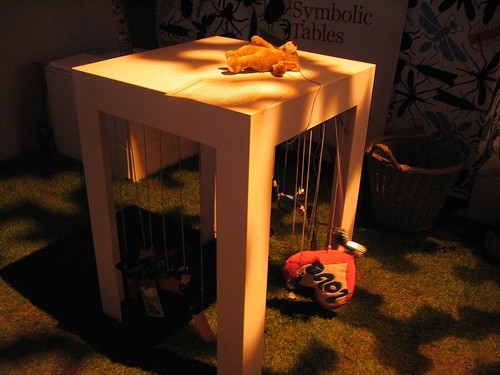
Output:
[{"left": 71, "top": 34, "right": 377, "bottom": 375}]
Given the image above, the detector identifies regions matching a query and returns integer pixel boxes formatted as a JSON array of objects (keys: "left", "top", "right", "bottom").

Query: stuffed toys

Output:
[
  {"left": 225, "top": 34, "right": 301, "bottom": 77},
  {"left": 282, "top": 249, "right": 355, "bottom": 309}
]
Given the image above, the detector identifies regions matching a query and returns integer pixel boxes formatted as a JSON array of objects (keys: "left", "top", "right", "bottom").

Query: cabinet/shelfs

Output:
[{"left": 44, "top": 52, "right": 202, "bottom": 183}]
